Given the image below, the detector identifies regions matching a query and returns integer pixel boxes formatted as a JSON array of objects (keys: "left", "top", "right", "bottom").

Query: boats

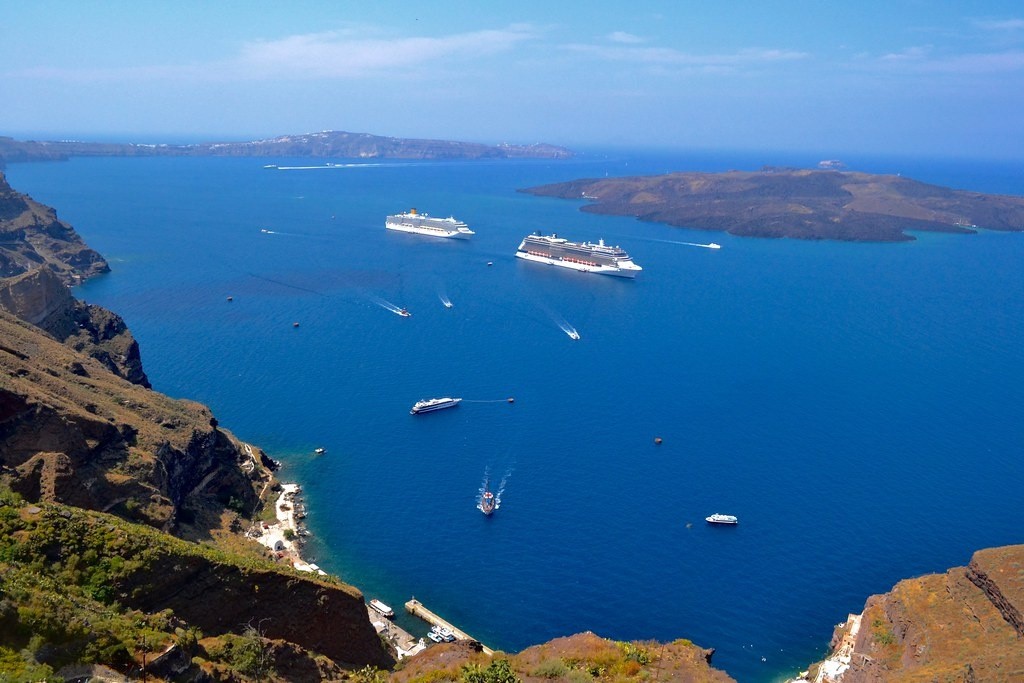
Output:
[
  {"left": 410, "top": 397, "right": 462, "bottom": 417},
  {"left": 482, "top": 491, "right": 495, "bottom": 515},
  {"left": 706, "top": 512, "right": 738, "bottom": 525}
]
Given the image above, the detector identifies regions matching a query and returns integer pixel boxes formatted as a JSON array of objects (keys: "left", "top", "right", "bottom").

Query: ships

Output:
[
  {"left": 386, "top": 208, "right": 476, "bottom": 239},
  {"left": 514, "top": 230, "right": 643, "bottom": 279}
]
